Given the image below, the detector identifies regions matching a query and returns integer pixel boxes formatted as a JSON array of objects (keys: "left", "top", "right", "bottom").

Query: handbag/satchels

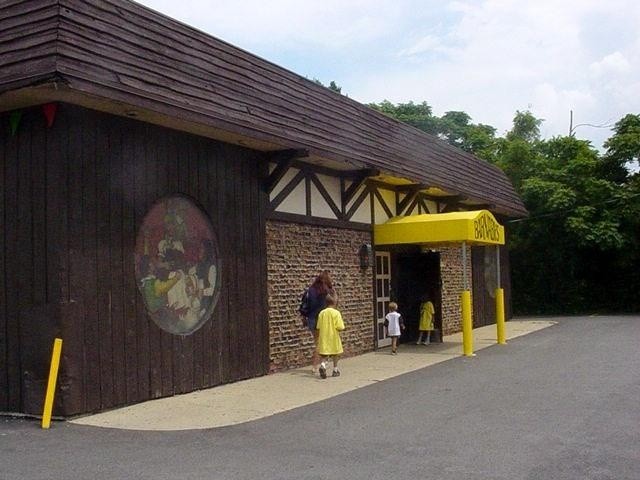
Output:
[{"left": 299, "top": 291, "right": 310, "bottom": 316}]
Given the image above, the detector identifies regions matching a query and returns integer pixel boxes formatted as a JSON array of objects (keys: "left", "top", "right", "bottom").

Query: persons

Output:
[
  {"left": 138, "top": 197, "right": 219, "bottom": 334},
  {"left": 300, "top": 270, "right": 335, "bottom": 370},
  {"left": 314, "top": 290, "right": 345, "bottom": 376},
  {"left": 383, "top": 301, "right": 405, "bottom": 355},
  {"left": 416, "top": 292, "right": 437, "bottom": 345}
]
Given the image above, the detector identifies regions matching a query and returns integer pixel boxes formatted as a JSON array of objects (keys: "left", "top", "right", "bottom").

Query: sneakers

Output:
[
  {"left": 416, "top": 341, "right": 430, "bottom": 345},
  {"left": 311, "top": 364, "right": 340, "bottom": 378},
  {"left": 391, "top": 347, "right": 398, "bottom": 355}
]
